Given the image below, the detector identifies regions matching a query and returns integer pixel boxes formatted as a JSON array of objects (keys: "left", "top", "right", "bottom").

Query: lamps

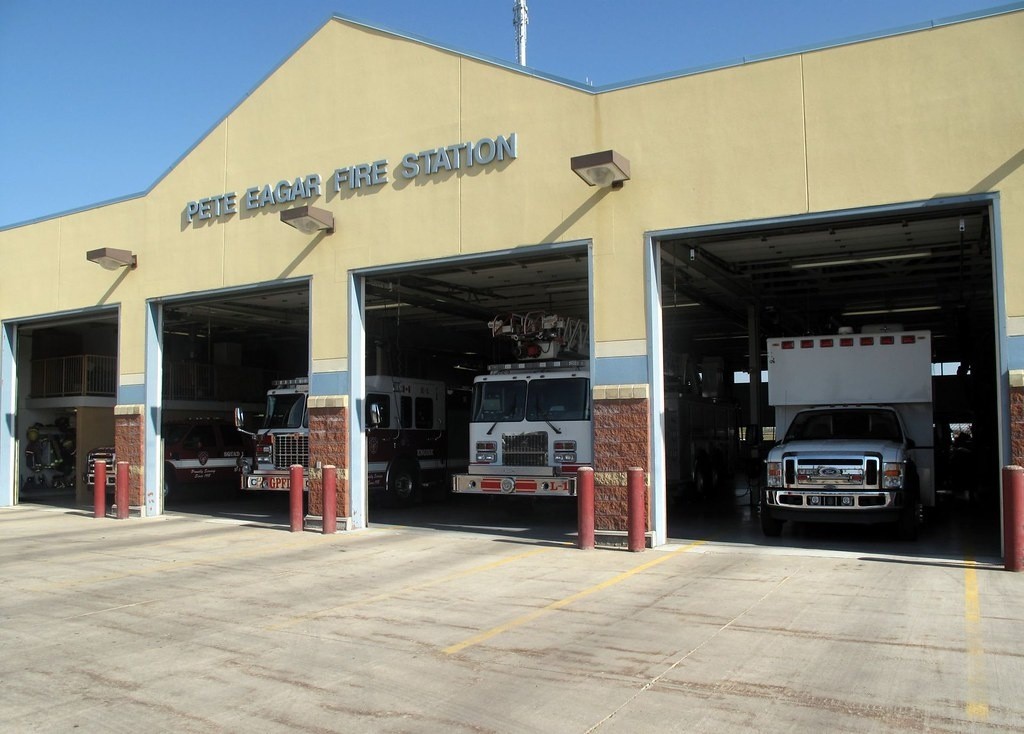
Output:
[
  {"left": 280, "top": 205, "right": 334, "bottom": 236},
  {"left": 87, "top": 247, "right": 135, "bottom": 272},
  {"left": 570, "top": 151, "right": 632, "bottom": 190}
]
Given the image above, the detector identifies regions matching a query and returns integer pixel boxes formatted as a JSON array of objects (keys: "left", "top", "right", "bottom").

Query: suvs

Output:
[{"left": 84, "top": 416, "right": 257, "bottom": 508}]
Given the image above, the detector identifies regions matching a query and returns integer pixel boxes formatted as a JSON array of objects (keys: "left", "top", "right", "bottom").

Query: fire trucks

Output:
[
  {"left": 453, "top": 310, "right": 705, "bottom": 496},
  {"left": 756, "top": 331, "right": 937, "bottom": 538},
  {"left": 232, "top": 374, "right": 500, "bottom": 509}
]
